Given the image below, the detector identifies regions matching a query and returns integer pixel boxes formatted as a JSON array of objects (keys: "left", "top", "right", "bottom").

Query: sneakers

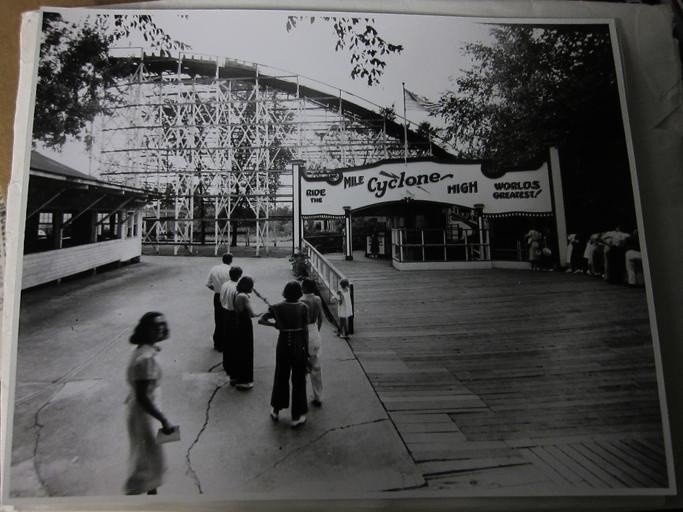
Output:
[
  {"left": 270, "top": 409, "right": 279, "bottom": 421},
  {"left": 230, "top": 378, "right": 253, "bottom": 391},
  {"left": 311, "top": 399, "right": 322, "bottom": 408},
  {"left": 292, "top": 415, "right": 307, "bottom": 427}
]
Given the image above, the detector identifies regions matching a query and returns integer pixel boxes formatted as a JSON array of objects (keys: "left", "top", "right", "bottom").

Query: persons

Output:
[
  {"left": 298, "top": 276, "right": 323, "bottom": 407},
  {"left": 124, "top": 312, "right": 173, "bottom": 496},
  {"left": 526, "top": 225, "right": 642, "bottom": 289},
  {"left": 370, "top": 232, "right": 380, "bottom": 259},
  {"left": 207, "top": 253, "right": 262, "bottom": 388},
  {"left": 335, "top": 279, "right": 353, "bottom": 340},
  {"left": 257, "top": 281, "right": 311, "bottom": 427}
]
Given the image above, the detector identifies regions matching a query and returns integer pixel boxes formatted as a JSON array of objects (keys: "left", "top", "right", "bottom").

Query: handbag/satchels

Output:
[{"left": 154, "top": 425, "right": 181, "bottom": 444}]
